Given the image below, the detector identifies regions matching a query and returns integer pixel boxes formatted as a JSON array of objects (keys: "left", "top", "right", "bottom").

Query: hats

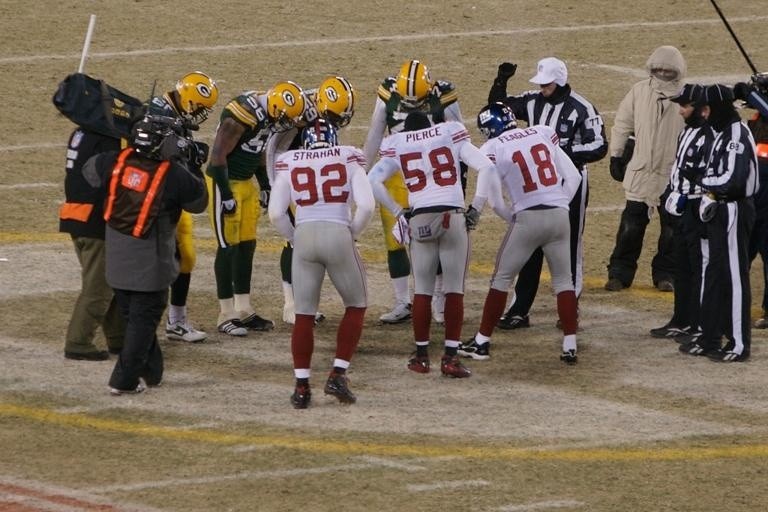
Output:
[
  {"left": 669, "top": 82, "right": 733, "bottom": 108},
  {"left": 529, "top": 57, "right": 568, "bottom": 87},
  {"left": 404, "top": 112, "right": 431, "bottom": 131}
]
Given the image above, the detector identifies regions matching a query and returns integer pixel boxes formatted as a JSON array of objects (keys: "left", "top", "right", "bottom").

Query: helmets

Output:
[
  {"left": 267, "top": 81, "right": 306, "bottom": 133},
  {"left": 477, "top": 102, "right": 518, "bottom": 139},
  {"left": 162, "top": 71, "right": 218, "bottom": 126},
  {"left": 396, "top": 60, "right": 430, "bottom": 108},
  {"left": 314, "top": 76, "right": 355, "bottom": 127},
  {"left": 301, "top": 119, "right": 339, "bottom": 149}
]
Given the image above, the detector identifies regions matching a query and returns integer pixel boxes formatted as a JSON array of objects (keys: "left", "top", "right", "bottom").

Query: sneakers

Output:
[
  {"left": 650, "top": 318, "right": 768, "bottom": 363},
  {"left": 290, "top": 382, "right": 312, "bottom": 410},
  {"left": 407, "top": 333, "right": 491, "bottom": 377},
  {"left": 325, "top": 372, "right": 356, "bottom": 404},
  {"left": 380, "top": 303, "right": 414, "bottom": 324},
  {"left": 166, "top": 318, "right": 207, "bottom": 342},
  {"left": 432, "top": 295, "right": 446, "bottom": 324},
  {"left": 217, "top": 310, "right": 327, "bottom": 336},
  {"left": 65, "top": 344, "right": 164, "bottom": 396},
  {"left": 657, "top": 280, "right": 674, "bottom": 292},
  {"left": 604, "top": 277, "right": 623, "bottom": 292},
  {"left": 496, "top": 314, "right": 530, "bottom": 329},
  {"left": 559, "top": 349, "right": 579, "bottom": 367}
]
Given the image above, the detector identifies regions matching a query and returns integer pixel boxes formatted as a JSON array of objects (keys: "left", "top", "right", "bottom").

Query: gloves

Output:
[
  {"left": 680, "top": 164, "right": 706, "bottom": 183},
  {"left": 397, "top": 207, "right": 413, "bottom": 221},
  {"left": 465, "top": 204, "right": 480, "bottom": 231},
  {"left": 622, "top": 132, "right": 636, "bottom": 165},
  {"left": 259, "top": 190, "right": 270, "bottom": 208},
  {"left": 610, "top": 156, "right": 625, "bottom": 182},
  {"left": 221, "top": 197, "right": 238, "bottom": 215},
  {"left": 498, "top": 62, "right": 517, "bottom": 82}
]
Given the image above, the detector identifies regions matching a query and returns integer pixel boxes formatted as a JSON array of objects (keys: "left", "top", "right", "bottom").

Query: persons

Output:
[
  {"left": 367, "top": 113, "right": 493, "bottom": 379},
  {"left": 53, "top": 71, "right": 219, "bottom": 397},
  {"left": 207, "top": 75, "right": 356, "bottom": 337},
  {"left": 488, "top": 56, "right": 609, "bottom": 329},
  {"left": 357, "top": 60, "right": 468, "bottom": 323},
  {"left": 456, "top": 100, "right": 584, "bottom": 364},
  {"left": 648, "top": 82, "right": 768, "bottom": 362},
  {"left": 268, "top": 120, "right": 377, "bottom": 409},
  {"left": 604, "top": 43, "right": 686, "bottom": 293}
]
